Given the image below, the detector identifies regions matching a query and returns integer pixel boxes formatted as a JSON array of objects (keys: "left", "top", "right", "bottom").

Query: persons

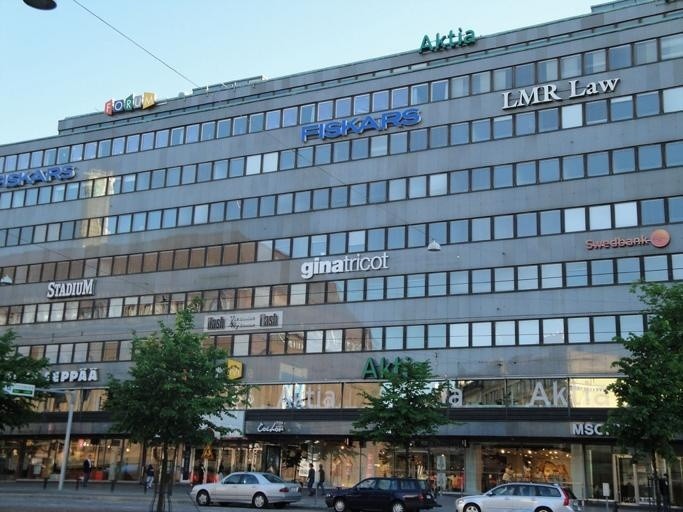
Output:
[
  {"left": 307, "top": 463, "right": 325, "bottom": 496},
  {"left": 218, "top": 460, "right": 224, "bottom": 480},
  {"left": 83, "top": 455, "right": 92, "bottom": 487},
  {"left": 146, "top": 464, "right": 155, "bottom": 488},
  {"left": 659, "top": 474, "right": 670, "bottom": 512},
  {"left": 198, "top": 463, "right": 205, "bottom": 484},
  {"left": 502, "top": 462, "right": 572, "bottom": 482}
]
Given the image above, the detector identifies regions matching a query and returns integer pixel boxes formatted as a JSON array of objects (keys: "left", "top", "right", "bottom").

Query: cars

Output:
[
  {"left": 455, "top": 481, "right": 583, "bottom": 512},
  {"left": 190, "top": 472, "right": 303, "bottom": 509},
  {"left": 324, "top": 476, "right": 436, "bottom": 512}
]
